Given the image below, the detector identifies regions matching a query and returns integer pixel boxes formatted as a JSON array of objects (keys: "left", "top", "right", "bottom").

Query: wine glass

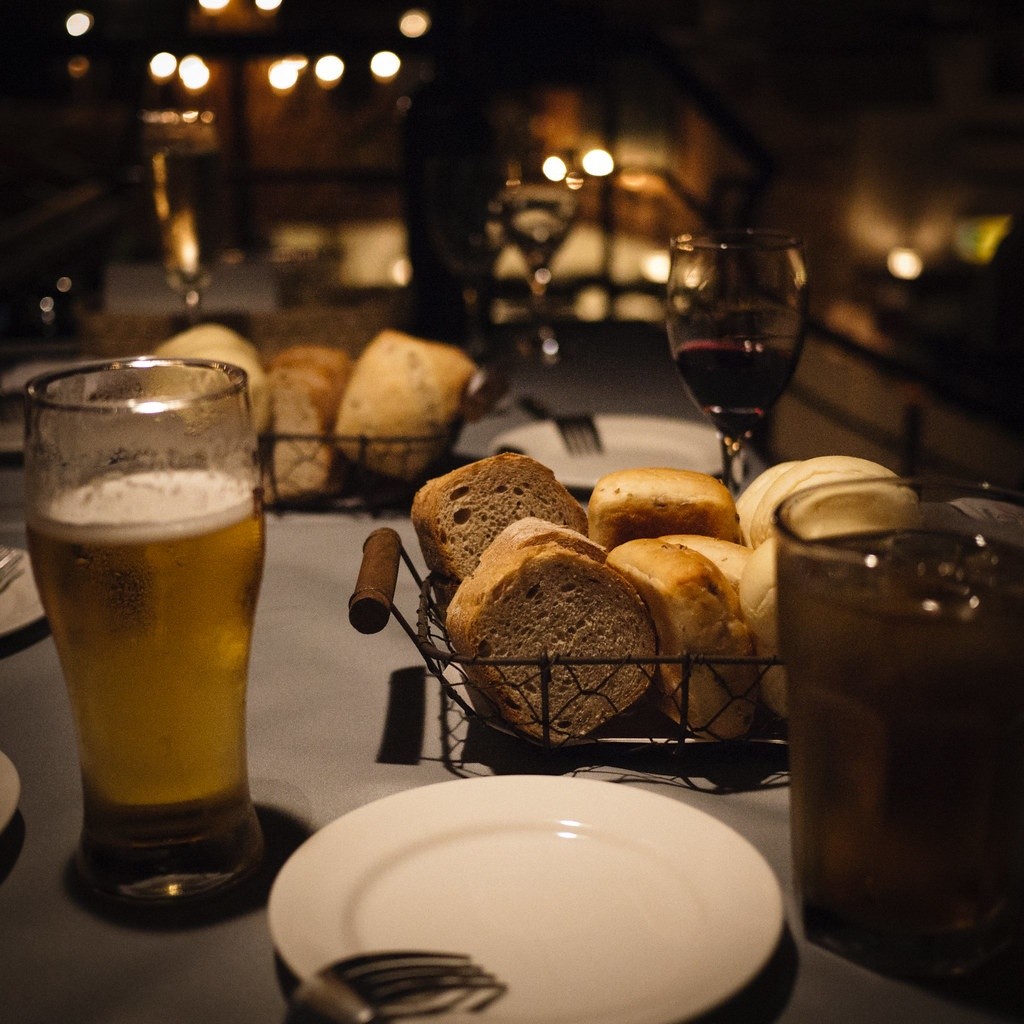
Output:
[{"left": 666, "top": 233, "right": 806, "bottom": 490}]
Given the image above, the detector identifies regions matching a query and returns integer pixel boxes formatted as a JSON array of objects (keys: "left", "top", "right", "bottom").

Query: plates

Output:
[
  {"left": 0, "top": 751, "right": 19, "bottom": 832},
  {"left": 491, "top": 416, "right": 729, "bottom": 493},
  {"left": 267, "top": 773, "right": 784, "bottom": 1021},
  {"left": 1, "top": 547, "right": 46, "bottom": 642}
]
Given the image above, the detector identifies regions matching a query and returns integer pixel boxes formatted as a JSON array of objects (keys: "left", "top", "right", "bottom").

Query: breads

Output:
[
  {"left": 408, "top": 444, "right": 920, "bottom": 740},
  {"left": 147, "top": 321, "right": 478, "bottom": 500}
]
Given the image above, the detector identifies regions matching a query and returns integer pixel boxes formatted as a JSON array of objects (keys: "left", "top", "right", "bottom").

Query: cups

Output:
[
  {"left": 775, "top": 478, "right": 1021, "bottom": 1011},
  {"left": 20, "top": 358, "right": 268, "bottom": 901}
]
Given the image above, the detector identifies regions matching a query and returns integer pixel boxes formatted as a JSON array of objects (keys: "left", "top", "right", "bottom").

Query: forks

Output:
[
  {"left": 520, "top": 393, "right": 601, "bottom": 458},
  {"left": 0, "top": 547, "right": 19, "bottom": 569},
  {"left": 280, "top": 951, "right": 507, "bottom": 1022}
]
